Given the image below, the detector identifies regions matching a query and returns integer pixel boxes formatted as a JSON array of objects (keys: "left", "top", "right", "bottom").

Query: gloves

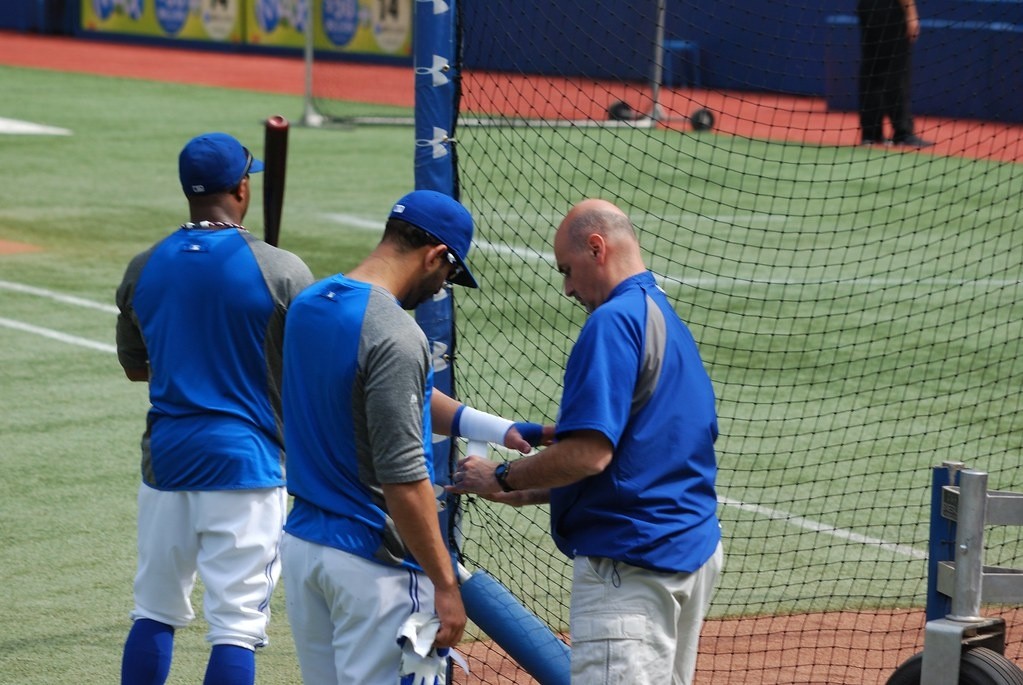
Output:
[{"left": 396, "top": 611, "right": 452, "bottom": 685}]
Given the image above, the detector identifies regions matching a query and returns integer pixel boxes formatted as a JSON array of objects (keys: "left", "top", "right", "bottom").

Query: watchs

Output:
[{"left": 495, "top": 460, "right": 513, "bottom": 492}]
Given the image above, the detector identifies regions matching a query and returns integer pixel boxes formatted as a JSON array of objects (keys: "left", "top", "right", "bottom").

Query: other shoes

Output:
[
  {"left": 862, "top": 138, "right": 893, "bottom": 144},
  {"left": 892, "top": 135, "right": 935, "bottom": 147}
]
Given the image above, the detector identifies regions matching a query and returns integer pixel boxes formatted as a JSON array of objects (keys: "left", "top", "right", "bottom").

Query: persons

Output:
[
  {"left": 279, "top": 189, "right": 478, "bottom": 685},
  {"left": 443, "top": 196, "right": 724, "bottom": 685},
  {"left": 112, "top": 133, "right": 319, "bottom": 685},
  {"left": 857, "top": 0, "right": 933, "bottom": 149}
]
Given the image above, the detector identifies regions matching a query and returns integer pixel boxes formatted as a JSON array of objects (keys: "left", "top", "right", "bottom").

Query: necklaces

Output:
[{"left": 181, "top": 219, "right": 248, "bottom": 231}]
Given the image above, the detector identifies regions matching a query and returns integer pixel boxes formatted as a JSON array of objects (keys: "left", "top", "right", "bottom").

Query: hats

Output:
[
  {"left": 387, "top": 189, "right": 480, "bottom": 290},
  {"left": 179, "top": 133, "right": 264, "bottom": 196}
]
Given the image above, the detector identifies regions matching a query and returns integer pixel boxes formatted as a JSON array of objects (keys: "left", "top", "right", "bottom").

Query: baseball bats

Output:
[{"left": 260, "top": 114, "right": 289, "bottom": 248}]
